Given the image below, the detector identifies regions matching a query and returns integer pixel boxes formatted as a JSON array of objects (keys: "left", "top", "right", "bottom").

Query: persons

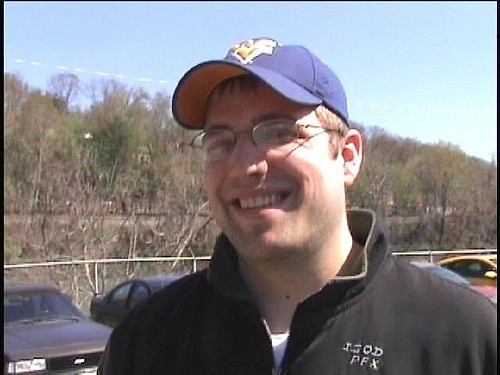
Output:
[{"left": 98, "top": 36, "right": 496, "bottom": 375}]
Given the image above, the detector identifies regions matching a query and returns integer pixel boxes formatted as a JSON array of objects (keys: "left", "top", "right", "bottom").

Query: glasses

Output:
[{"left": 186, "top": 119, "right": 347, "bottom": 163}]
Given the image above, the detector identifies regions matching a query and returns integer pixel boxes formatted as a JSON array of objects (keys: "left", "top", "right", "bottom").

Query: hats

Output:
[{"left": 171, "top": 37, "right": 350, "bottom": 131}]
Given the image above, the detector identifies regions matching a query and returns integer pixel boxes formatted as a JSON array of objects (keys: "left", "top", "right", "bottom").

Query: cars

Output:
[
  {"left": 89, "top": 275, "right": 185, "bottom": 329},
  {"left": 3, "top": 281, "right": 115, "bottom": 375},
  {"left": 437, "top": 254, "right": 498, "bottom": 287},
  {"left": 407, "top": 261, "right": 497, "bottom": 306}
]
